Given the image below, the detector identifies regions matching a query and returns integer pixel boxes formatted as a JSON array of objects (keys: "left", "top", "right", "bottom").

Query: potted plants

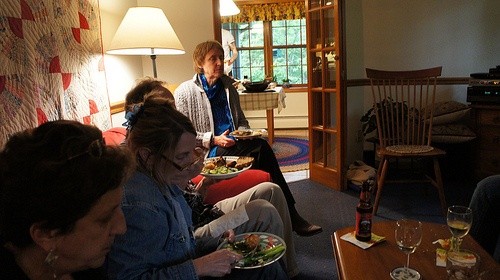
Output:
[
  {"left": 268, "top": 75, "right": 277, "bottom": 87},
  {"left": 282, "top": 79, "right": 293, "bottom": 88}
]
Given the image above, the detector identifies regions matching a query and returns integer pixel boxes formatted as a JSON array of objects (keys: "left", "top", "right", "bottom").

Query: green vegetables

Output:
[
  {"left": 207, "top": 166, "right": 233, "bottom": 174},
  {"left": 222, "top": 240, "right": 285, "bottom": 266}
]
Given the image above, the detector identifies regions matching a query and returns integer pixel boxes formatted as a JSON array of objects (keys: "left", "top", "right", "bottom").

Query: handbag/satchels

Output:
[{"left": 360, "top": 97, "right": 408, "bottom": 136}]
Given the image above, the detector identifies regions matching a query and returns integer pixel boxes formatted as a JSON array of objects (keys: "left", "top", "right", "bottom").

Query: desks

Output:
[{"left": 237, "top": 87, "right": 287, "bottom": 146}]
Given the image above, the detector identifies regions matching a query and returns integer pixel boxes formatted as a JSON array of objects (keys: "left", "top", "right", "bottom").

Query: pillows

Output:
[
  {"left": 420, "top": 100, "right": 472, "bottom": 126},
  {"left": 425, "top": 123, "right": 477, "bottom": 142}
]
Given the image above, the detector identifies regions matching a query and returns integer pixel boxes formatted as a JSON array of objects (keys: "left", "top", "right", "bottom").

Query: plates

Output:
[
  {"left": 200, "top": 156, "right": 253, "bottom": 179},
  {"left": 217, "top": 232, "right": 286, "bottom": 269},
  {"left": 229, "top": 130, "right": 263, "bottom": 139}
]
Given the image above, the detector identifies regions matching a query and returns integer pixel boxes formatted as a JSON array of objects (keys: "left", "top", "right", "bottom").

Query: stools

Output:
[{"left": 361, "top": 130, "right": 415, "bottom": 178}]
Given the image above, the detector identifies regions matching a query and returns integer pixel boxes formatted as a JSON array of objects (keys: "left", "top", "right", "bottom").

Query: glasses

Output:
[
  {"left": 136, "top": 147, "right": 202, "bottom": 172},
  {"left": 23, "top": 138, "right": 105, "bottom": 176}
]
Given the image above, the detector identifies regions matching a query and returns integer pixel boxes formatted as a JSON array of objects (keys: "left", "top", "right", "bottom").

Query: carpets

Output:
[{"left": 258, "top": 127, "right": 310, "bottom": 173}]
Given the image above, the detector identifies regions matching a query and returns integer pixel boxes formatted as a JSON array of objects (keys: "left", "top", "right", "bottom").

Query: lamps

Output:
[
  {"left": 105, "top": 6, "right": 186, "bottom": 79},
  {"left": 219, "top": 0, "right": 240, "bottom": 16}
]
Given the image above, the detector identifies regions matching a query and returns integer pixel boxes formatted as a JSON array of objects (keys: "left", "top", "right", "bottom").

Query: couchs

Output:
[{"left": 101, "top": 127, "right": 271, "bottom": 207}]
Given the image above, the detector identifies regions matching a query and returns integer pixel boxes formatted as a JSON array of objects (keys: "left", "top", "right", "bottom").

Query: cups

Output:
[
  {"left": 446, "top": 249, "right": 480, "bottom": 280},
  {"left": 270, "top": 84, "right": 275, "bottom": 90},
  {"left": 390, "top": 267, "right": 420, "bottom": 280}
]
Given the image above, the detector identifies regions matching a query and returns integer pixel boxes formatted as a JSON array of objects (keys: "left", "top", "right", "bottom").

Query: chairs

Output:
[{"left": 365, "top": 66, "right": 448, "bottom": 223}]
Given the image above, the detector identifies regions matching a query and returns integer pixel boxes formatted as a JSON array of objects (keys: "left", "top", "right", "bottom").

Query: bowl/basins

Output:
[{"left": 243, "top": 82, "right": 270, "bottom": 92}]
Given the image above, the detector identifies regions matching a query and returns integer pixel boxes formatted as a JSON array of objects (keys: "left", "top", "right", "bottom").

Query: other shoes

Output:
[
  {"left": 292, "top": 224, "right": 323, "bottom": 236},
  {"left": 291, "top": 272, "right": 316, "bottom": 280}
]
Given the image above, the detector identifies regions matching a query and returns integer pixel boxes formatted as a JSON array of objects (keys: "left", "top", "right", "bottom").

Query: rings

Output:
[{"left": 233, "top": 255, "right": 236, "bottom": 262}]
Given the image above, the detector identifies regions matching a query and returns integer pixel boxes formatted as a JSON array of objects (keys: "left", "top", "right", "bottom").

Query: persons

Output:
[
  {"left": 468, "top": 174, "right": 500, "bottom": 256},
  {"left": 126, "top": 79, "right": 299, "bottom": 280},
  {"left": 104, "top": 90, "right": 243, "bottom": 280},
  {"left": 173, "top": 41, "right": 322, "bottom": 235},
  {"left": 222, "top": 28, "right": 238, "bottom": 79},
  {"left": 0, "top": 120, "right": 127, "bottom": 280}
]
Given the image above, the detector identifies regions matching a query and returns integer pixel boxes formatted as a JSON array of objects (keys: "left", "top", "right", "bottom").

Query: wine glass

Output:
[
  {"left": 390, "top": 219, "right": 423, "bottom": 266},
  {"left": 447, "top": 206, "right": 472, "bottom": 250}
]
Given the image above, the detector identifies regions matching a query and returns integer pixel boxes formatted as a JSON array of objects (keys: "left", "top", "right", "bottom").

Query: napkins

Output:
[{"left": 340, "top": 231, "right": 385, "bottom": 250}]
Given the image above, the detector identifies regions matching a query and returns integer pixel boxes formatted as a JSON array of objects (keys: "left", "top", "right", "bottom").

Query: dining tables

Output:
[{"left": 330, "top": 220, "right": 500, "bottom": 280}]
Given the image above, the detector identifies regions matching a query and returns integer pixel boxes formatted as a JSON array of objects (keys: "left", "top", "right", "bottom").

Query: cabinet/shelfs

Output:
[{"left": 468, "top": 102, "right": 500, "bottom": 180}]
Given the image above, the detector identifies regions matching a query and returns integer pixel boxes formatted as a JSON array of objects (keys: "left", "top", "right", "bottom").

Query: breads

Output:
[
  {"left": 233, "top": 131, "right": 252, "bottom": 136},
  {"left": 234, "top": 156, "right": 254, "bottom": 168}
]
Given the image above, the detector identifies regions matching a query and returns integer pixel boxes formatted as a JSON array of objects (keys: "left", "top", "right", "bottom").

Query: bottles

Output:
[
  {"left": 242, "top": 76, "right": 250, "bottom": 90},
  {"left": 355, "top": 182, "right": 373, "bottom": 242}
]
